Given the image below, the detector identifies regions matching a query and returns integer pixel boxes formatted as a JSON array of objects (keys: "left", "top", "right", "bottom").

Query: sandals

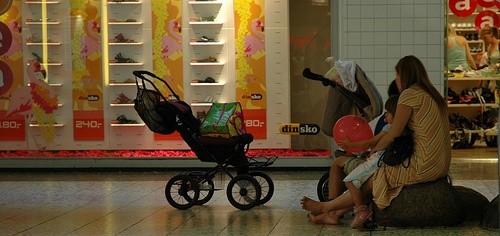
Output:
[
  {"left": 109, "top": 33, "right": 134, "bottom": 43},
  {"left": 112, "top": 92, "right": 135, "bottom": 104},
  {"left": 350, "top": 204, "right": 373, "bottom": 229},
  {"left": 109, "top": 52, "right": 137, "bottom": 63},
  {"left": 28, "top": 52, "right": 58, "bottom": 63},
  {"left": 111, "top": 114, "right": 137, "bottom": 124},
  {"left": 26, "top": 36, "right": 58, "bottom": 43}
]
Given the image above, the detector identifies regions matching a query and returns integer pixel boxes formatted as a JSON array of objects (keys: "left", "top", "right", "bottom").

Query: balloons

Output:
[{"left": 332, "top": 115, "right": 375, "bottom": 154}]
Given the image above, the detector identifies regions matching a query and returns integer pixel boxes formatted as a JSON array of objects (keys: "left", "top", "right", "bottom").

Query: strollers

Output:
[
  {"left": 133, "top": 69, "right": 278, "bottom": 211},
  {"left": 301, "top": 67, "right": 387, "bottom": 202}
]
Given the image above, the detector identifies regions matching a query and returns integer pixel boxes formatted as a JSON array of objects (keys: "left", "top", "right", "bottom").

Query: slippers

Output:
[
  {"left": 109, "top": 19, "right": 136, "bottom": 22},
  {"left": 190, "top": 15, "right": 215, "bottom": 22},
  {"left": 191, "top": 35, "right": 213, "bottom": 42},
  {"left": 32, "top": 120, "right": 58, "bottom": 124},
  {"left": 27, "top": 18, "right": 57, "bottom": 22},
  {"left": 192, "top": 77, "right": 218, "bottom": 83},
  {"left": 114, "top": 0, "right": 139, "bottom": 2},
  {"left": 191, "top": 97, "right": 217, "bottom": 103},
  {"left": 109, "top": 78, "right": 136, "bottom": 83},
  {"left": 191, "top": 56, "right": 216, "bottom": 62}
]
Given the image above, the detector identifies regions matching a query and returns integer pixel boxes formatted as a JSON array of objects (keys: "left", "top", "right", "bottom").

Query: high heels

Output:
[{"left": 467, "top": 33, "right": 478, "bottom": 40}]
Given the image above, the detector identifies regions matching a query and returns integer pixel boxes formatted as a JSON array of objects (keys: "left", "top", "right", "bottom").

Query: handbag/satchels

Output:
[
  {"left": 199, "top": 101, "right": 247, "bottom": 149},
  {"left": 132, "top": 76, "right": 176, "bottom": 135},
  {"left": 377, "top": 128, "right": 414, "bottom": 168},
  {"left": 446, "top": 86, "right": 498, "bottom": 148}
]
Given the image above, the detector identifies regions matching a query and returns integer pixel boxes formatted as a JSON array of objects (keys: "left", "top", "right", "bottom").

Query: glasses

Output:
[{"left": 450, "top": 70, "right": 462, "bottom": 73}]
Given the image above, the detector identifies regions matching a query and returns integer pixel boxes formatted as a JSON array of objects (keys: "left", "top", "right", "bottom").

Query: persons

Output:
[
  {"left": 445, "top": 22, "right": 477, "bottom": 71},
  {"left": 480, "top": 26, "right": 500, "bottom": 72},
  {"left": 300, "top": 55, "right": 452, "bottom": 229}
]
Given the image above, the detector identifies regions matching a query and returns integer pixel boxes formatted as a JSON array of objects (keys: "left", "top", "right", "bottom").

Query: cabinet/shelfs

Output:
[
  {"left": 189, "top": 0, "right": 225, "bottom": 108},
  {"left": 446, "top": 72, "right": 500, "bottom": 151},
  {"left": 107, "top": 1, "right": 147, "bottom": 127},
  {"left": 25, "top": 0, "right": 64, "bottom": 127}
]
